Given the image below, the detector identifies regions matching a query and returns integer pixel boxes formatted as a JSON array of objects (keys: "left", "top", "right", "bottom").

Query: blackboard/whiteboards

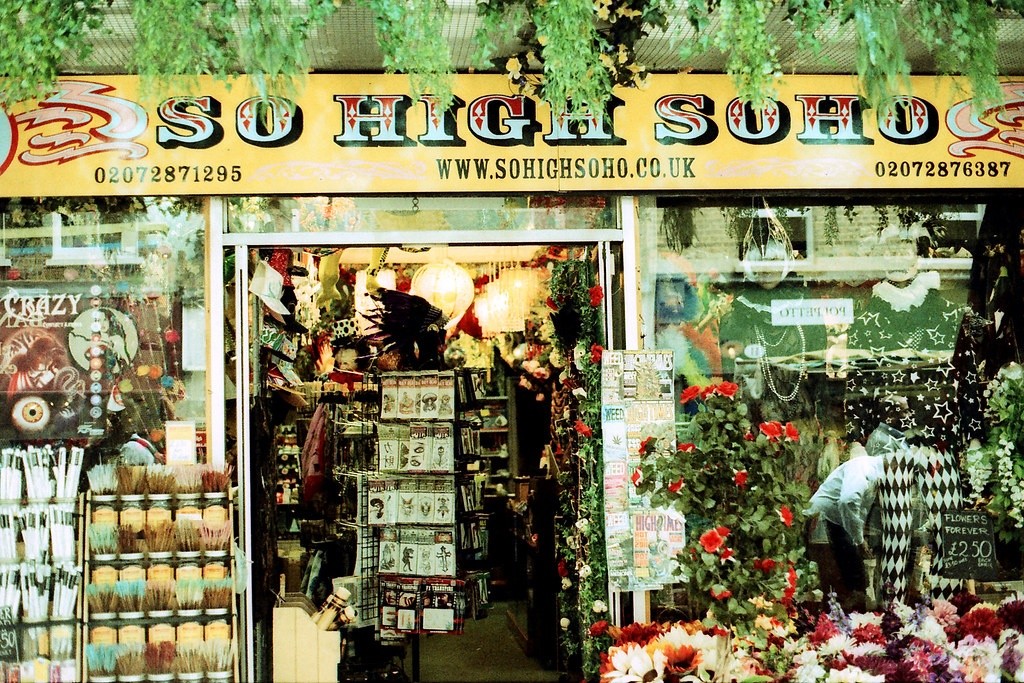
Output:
[{"left": 941, "top": 510, "right": 998, "bottom": 579}]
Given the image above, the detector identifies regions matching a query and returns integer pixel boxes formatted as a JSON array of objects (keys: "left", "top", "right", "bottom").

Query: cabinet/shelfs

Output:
[
  {"left": 83, "top": 487, "right": 240, "bottom": 683},
  {"left": 376, "top": 369, "right": 490, "bottom": 635},
  {"left": 0, "top": 493, "right": 83, "bottom": 683}
]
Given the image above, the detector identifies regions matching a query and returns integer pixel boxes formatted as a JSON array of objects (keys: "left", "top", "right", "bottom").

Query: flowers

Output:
[{"left": 517, "top": 246, "right": 1024, "bottom": 683}]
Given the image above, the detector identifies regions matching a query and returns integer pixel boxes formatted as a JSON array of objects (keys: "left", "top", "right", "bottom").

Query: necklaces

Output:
[{"left": 752, "top": 322, "right": 808, "bottom": 403}]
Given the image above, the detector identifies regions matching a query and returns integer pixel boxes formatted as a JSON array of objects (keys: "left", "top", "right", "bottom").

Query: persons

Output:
[
  {"left": 718, "top": 230, "right": 828, "bottom": 377},
  {"left": 801, "top": 451, "right": 920, "bottom": 612},
  {"left": 864, "top": 394, "right": 921, "bottom": 617}
]
